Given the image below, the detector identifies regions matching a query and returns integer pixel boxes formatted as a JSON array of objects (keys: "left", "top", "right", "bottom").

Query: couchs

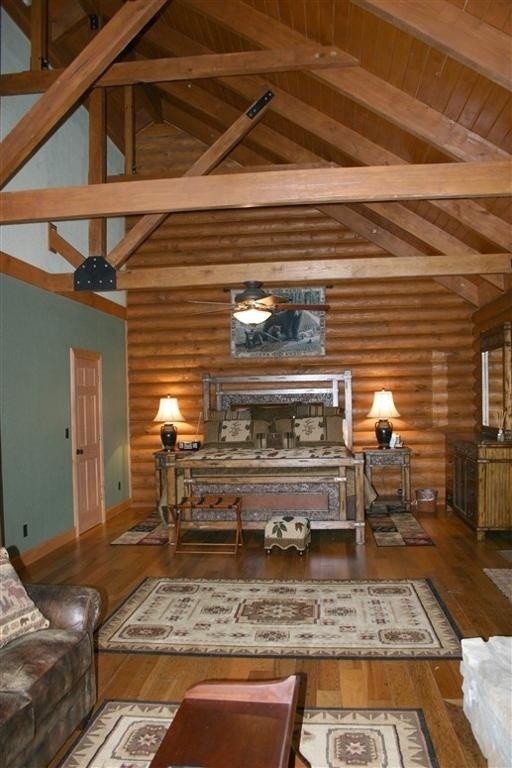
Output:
[{"left": 0, "top": 544, "right": 100, "bottom": 768}]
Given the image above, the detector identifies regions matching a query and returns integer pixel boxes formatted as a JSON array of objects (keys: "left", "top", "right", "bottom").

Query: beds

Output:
[{"left": 167, "top": 368, "right": 366, "bottom": 545}]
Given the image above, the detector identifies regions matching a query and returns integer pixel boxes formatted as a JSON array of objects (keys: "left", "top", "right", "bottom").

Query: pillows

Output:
[
  {"left": 205, "top": 407, "right": 273, "bottom": 449},
  {"left": 0, "top": 549, "right": 52, "bottom": 649},
  {"left": 273, "top": 404, "right": 346, "bottom": 446}
]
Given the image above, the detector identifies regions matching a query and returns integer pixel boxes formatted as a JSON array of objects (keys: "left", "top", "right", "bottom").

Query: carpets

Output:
[
  {"left": 497, "top": 549, "right": 511, "bottom": 563},
  {"left": 366, "top": 510, "right": 436, "bottom": 550},
  {"left": 98, "top": 577, "right": 470, "bottom": 662},
  {"left": 482, "top": 567, "right": 512, "bottom": 605},
  {"left": 58, "top": 697, "right": 442, "bottom": 767},
  {"left": 111, "top": 508, "right": 174, "bottom": 546}
]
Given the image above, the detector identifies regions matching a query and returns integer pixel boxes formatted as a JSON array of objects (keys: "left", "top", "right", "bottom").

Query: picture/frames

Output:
[{"left": 229, "top": 287, "right": 327, "bottom": 358}]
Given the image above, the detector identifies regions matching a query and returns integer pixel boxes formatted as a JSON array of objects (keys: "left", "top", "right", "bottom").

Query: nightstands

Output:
[
  {"left": 362, "top": 447, "right": 412, "bottom": 513},
  {"left": 152, "top": 452, "right": 186, "bottom": 504}
]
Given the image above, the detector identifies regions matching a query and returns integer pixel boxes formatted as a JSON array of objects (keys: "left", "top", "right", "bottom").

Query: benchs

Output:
[{"left": 150, "top": 677, "right": 312, "bottom": 768}]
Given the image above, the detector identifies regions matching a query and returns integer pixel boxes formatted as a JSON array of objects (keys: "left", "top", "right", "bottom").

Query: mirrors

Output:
[{"left": 474, "top": 321, "right": 512, "bottom": 437}]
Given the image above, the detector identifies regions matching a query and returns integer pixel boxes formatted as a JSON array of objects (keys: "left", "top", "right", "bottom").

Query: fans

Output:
[{"left": 181, "top": 281, "right": 330, "bottom": 319}]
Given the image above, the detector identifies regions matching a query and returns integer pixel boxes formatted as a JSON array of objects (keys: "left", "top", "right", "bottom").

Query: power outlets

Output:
[
  {"left": 179, "top": 441, "right": 200, "bottom": 450},
  {"left": 118, "top": 481, "right": 121, "bottom": 490},
  {"left": 65, "top": 428, "right": 70, "bottom": 439},
  {"left": 23, "top": 524, "right": 28, "bottom": 537}
]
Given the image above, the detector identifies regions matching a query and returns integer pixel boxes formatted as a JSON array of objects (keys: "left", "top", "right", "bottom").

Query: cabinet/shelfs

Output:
[{"left": 439, "top": 427, "right": 508, "bottom": 542}]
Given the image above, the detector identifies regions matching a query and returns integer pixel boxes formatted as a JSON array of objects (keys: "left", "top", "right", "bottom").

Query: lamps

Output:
[
  {"left": 367, "top": 387, "right": 402, "bottom": 450},
  {"left": 151, "top": 393, "right": 186, "bottom": 449}
]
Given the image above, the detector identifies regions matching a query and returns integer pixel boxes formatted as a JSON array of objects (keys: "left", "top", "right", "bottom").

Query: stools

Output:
[
  {"left": 175, "top": 495, "right": 243, "bottom": 553},
  {"left": 263, "top": 516, "right": 314, "bottom": 555}
]
